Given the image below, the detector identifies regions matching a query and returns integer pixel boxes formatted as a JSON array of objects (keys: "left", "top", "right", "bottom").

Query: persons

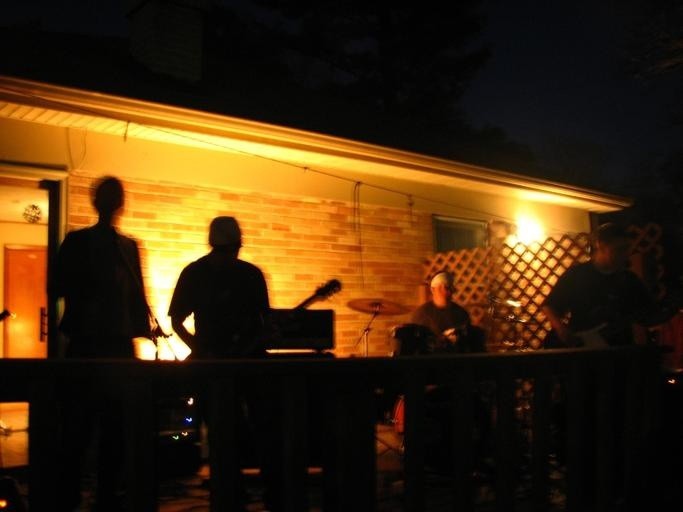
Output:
[
  {"left": 40, "top": 171, "right": 151, "bottom": 363},
  {"left": 413, "top": 268, "right": 469, "bottom": 349},
  {"left": 540, "top": 221, "right": 682, "bottom": 348},
  {"left": 165, "top": 214, "right": 271, "bottom": 359}
]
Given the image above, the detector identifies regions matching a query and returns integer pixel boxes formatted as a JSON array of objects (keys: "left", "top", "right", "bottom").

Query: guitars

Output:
[
  {"left": 565, "top": 286, "right": 681, "bottom": 370},
  {"left": 187, "top": 279, "right": 342, "bottom": 383}
]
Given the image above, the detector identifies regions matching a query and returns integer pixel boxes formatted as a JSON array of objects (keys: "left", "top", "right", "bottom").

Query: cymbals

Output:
[{"left": 346, "top": 297, "right": 411, "bottom": 317}]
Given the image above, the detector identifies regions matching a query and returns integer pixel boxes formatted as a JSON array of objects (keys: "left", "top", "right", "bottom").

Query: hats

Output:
[{"left": 592, "top": 222, "right": 638, "bottom": 240}]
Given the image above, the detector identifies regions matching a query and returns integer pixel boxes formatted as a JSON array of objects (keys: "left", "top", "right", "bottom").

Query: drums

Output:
[
  {"left": 448, "top": 327, "right": 486, "bottom": 362},
  {"left": 395, "top": 325, "right": 435, "bottom": 357},
  {"left": 390, "top": 385, "right": 496, "bottom": 476}
]
